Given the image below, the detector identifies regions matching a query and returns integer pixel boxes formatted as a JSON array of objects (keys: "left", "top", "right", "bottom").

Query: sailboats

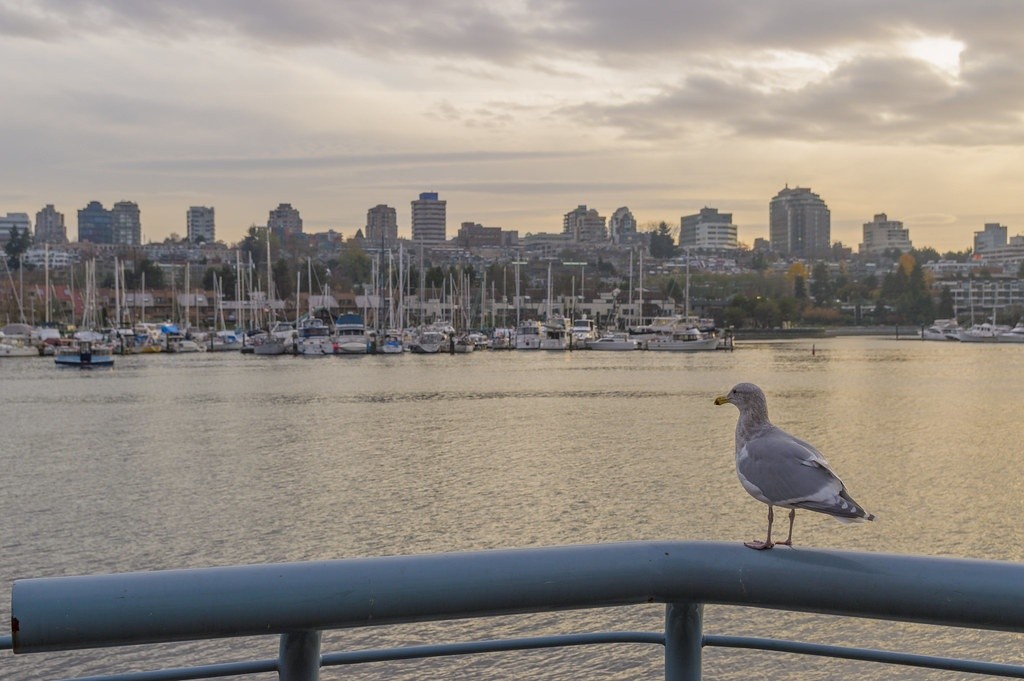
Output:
[
  {"left": 0, "top": 241, "right": 734, "bottom": 367},
  {"left": 917, "top": 281, "right": 1024, "bottom": 343}
]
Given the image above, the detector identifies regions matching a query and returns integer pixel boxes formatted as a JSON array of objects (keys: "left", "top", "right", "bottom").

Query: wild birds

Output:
[{"left": 713, "top": 383, "right": 881, "bottom": 551}]
